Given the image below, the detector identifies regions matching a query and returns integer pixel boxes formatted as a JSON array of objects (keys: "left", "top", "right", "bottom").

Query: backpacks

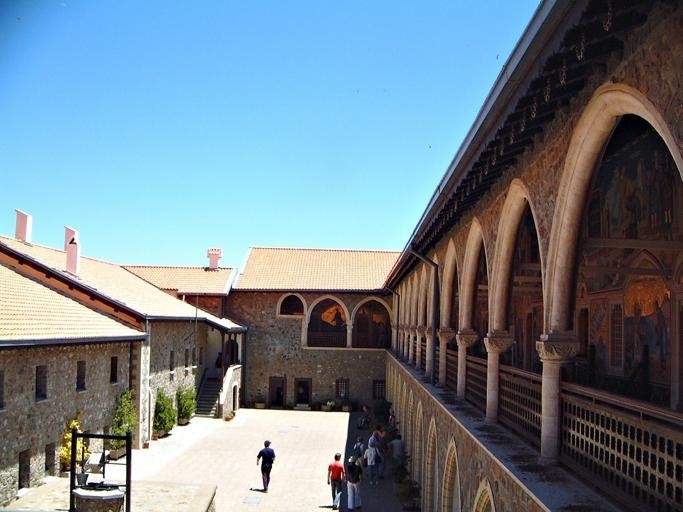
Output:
[{"left": 353, "top": 443, "right": 362, "bottom": 459}]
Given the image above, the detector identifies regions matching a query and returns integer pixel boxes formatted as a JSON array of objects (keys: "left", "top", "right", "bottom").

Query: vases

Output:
[
  {"left": 152, "top": 433, "right": 158, "bottom": 440},
  {"left": 144, "top": 442, "right": 149, "bottom": 448}
]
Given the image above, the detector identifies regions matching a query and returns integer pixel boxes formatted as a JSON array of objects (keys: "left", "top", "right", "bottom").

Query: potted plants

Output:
[
  {"left": 225, "top": 411, "right": 235, "bottom": 421},
  {"left": 153, "top": 383, "right": 198, "bottom": 437},
  {"left": 58, "top": 409, "right": 90, "bottom": 479},
  {"left": 321, "top": 400, "right": 336, "bottom": 411},
  {"left": 108, "top": 389, "right": 136, "bottom": 459},
  {"left": 254, "top": 394, "right": 266, "bottom": 408}
]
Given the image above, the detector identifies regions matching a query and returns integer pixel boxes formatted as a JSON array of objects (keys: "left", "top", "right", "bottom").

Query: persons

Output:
[
  {"left": 384, "top": 403, "right": 394, "bottom": 425},
  {"left": 214, "top": 351, "right": 223, "bottom": 384},
  {"left": 344, "top": 455, "right": 362, "bottom": 512},
  {"left": 375, "top": 423, "right": 387, "bottom": 441},
  {"left": 353, "top": 436, "right": 363, "bottom": 457},
  {"left": 256, "top": 440, "right": 274, "bottom": 492},
  {"left": 356, "top": 403, "right": 370, "bottom": 429},
  {"left": 223, "top": 350, "right": 230, "bottom": 375},
  {"left": 326, "top": 452, "right": 344, "bottom": 509},
  {"left": 368, "top": 431, "right": 379, "bottom": 445},
  {"left": 363, "top": 443, "right": 384, "bottom": 484},
  {"left": 387, "top": 434, "right": 403, "bottom": 471}
]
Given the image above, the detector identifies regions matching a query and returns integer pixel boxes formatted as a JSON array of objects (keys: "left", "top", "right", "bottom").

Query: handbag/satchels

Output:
[{"left": 374, "top": 447, "right": 382, "bottom": 464}]
